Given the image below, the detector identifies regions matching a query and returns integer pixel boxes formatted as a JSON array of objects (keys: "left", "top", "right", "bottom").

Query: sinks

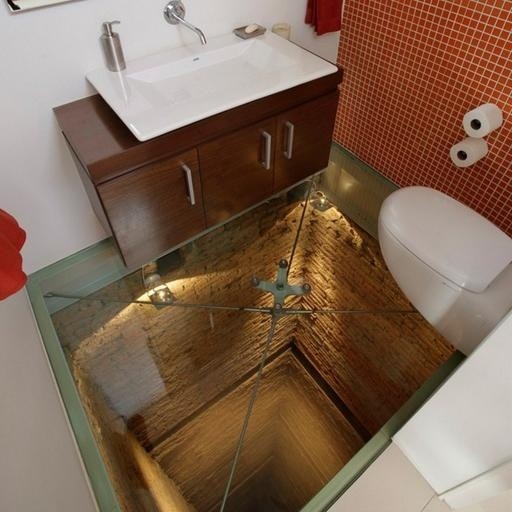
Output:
[{"left": 82, "top": 29, "right": 340, "bottom": 141}]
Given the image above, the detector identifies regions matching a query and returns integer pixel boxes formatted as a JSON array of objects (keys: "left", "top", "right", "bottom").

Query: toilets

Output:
[{"left": 378, "top": 187, "right": 512, "bottom": 356}]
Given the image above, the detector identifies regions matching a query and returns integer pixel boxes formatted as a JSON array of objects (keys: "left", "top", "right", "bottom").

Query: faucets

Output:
[{"left": 162, "top": 0, "right": 207, "bottom": 46}]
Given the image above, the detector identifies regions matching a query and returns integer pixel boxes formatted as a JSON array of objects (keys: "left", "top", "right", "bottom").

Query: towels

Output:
[{"left": 303, "top": 0, "right": 345, "bottom": 36}]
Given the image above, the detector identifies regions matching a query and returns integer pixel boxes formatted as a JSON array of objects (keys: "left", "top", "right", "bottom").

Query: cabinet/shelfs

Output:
[
  {"left": 96, "top": 149, "right": 208, "bottom": 269},
  {"left": 197, "top": 89, "right": 342, "bottom": 231}
]
